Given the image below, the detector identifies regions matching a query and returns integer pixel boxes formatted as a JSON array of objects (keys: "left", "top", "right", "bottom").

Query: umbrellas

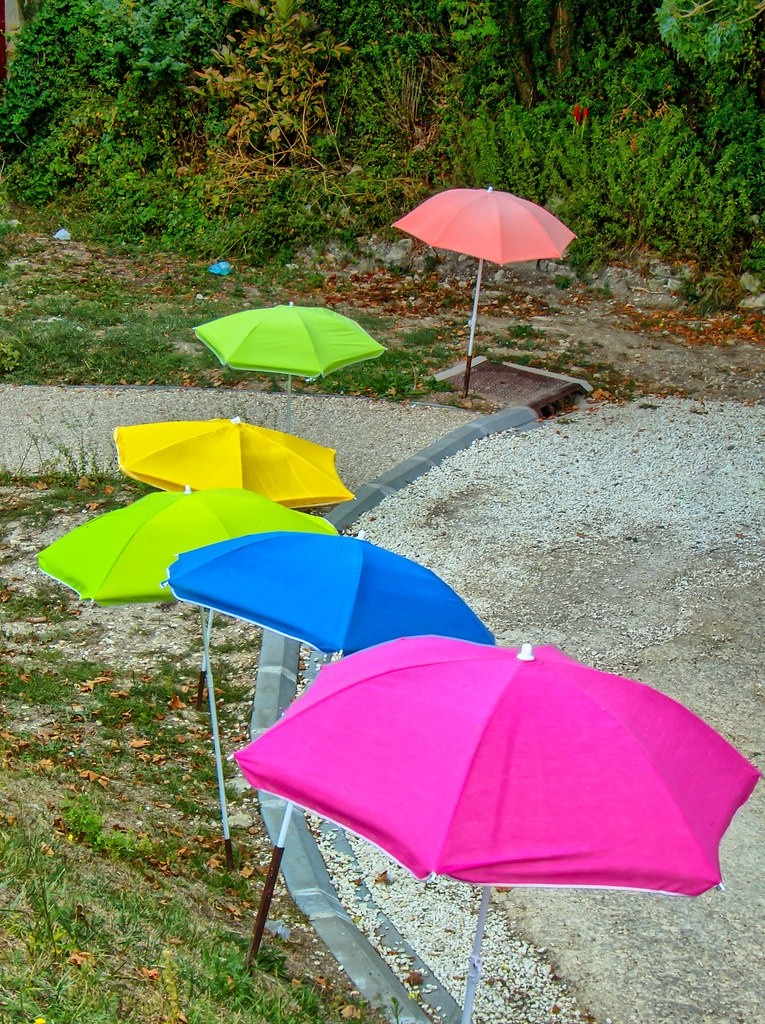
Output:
[
  {"left": 114, "top": 416, "right": 356, "bottom": 712},
  {"left": 34, "top": 486, "right": 340, "bottom": 870},
  {"left": 391, "top": 187, "right": 578, "bottom": 398},
  {"left": 233, "top": 634, "right": 762, "bottom": 1024},
  {"left": 160, "top": 531, "right": 495, "bottom": 963},
  {"left": 191, "top": 302, "right": 387, "bottom": 436}
]
